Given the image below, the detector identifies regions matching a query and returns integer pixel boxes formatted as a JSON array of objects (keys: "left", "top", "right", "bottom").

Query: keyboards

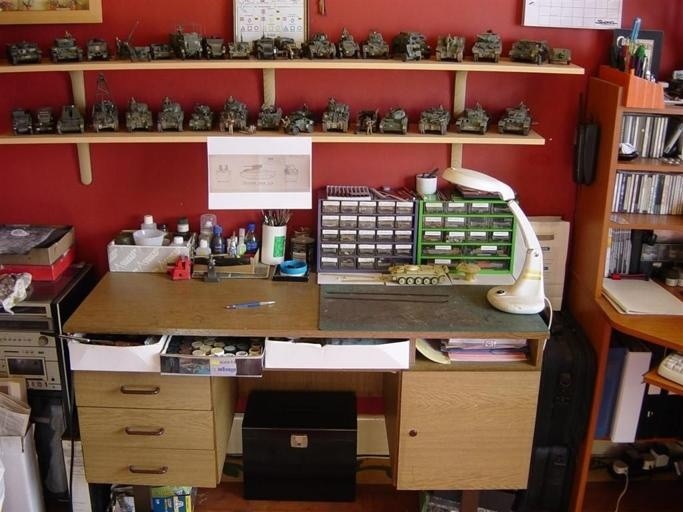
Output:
[{"left": 657, "top": 352, "right": 683, "bottom": 386}]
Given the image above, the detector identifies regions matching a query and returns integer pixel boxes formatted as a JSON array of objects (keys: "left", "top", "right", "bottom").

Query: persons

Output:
[
  {"left": 115, "top": 37, "right": 121, "bottom": 48},
  {"left": 130, "top": 97, "right": 136, "bottom": 104},
  {"left": 446, "top": 33, "right": 452, "bottom": 45},
  {"left": 421, "top": 119, "right": 426, "bottom": 133},
  {"left": 441, "top": 119, "right": 447, "bottom": 133},
  {"left": 65, "top": 31, "right": 71, "bottom": 39},
  {"left": 401, "top": 118, "right": 408, "bottom": 134},
  {"left": 366, "top": 118, "right": 374, "bottom": 133},
  {"left": 535, "top": 45, "right": 543, "bottom": 65},
  {"left": 165, "top": 97, "right": 171, "bottom": 105},
  {"left": 228, "top": 118, "right": 236, "bottom": 134}
]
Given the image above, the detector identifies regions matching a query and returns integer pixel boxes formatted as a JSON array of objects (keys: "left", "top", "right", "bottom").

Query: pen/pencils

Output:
[
  {"left": 261, "top": 209, "right": 292, "bottom": 226},
  {"left": 225, "top": 301, "right": 275, "bottom": 309},
  {"left": 421, "top": 168, "right": 439, "bottom": 178}
]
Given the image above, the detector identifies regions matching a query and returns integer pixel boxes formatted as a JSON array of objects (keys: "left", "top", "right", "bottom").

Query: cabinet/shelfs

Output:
[{"left": 555, "top": 66, "right": 681, "bottom": 511}]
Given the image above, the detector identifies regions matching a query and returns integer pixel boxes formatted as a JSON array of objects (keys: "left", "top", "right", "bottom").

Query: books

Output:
[{"left": 604, "top": 115, "right": 683, "bottom": 278}]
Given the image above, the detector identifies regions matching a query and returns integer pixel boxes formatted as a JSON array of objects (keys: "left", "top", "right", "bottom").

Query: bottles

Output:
[
  {"left": 141, "top": 215, "right": 190, "bottom": 246},
  {"left": 289, "top": 226, "right": 315, "bottom": 269},
  {"left": 195, "top": 214, "right": 259, "bottom": 258}
]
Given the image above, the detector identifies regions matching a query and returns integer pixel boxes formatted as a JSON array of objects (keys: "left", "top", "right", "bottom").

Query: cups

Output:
[
  {"left": 416, "top": 174, "right": 437, "bottom": 194},
  {"left": 131, "top": 229, "right": 163, "bottom": 253}
]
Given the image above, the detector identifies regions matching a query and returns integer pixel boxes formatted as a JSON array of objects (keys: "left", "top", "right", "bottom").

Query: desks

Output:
[{"left": 63, "top": 255, "right": 552, "bottom": 512}]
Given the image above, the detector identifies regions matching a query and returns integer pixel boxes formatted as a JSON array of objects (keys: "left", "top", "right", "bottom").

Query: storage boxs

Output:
[{"left": 241, "top": 389, "right": 358, "bottom": 504}]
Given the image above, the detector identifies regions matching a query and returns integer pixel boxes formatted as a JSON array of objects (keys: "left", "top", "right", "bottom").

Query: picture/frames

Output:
[
  {"left": 1, "top": 0, "right": 103, "bottom": 26},
  {"left": 611, "top": 29, "right": 664, "bottom": 83},
  {"left": 232, "top": 0, "right": 311, "bottom": 46}
]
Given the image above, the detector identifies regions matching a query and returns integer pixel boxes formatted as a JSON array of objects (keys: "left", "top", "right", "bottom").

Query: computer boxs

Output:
[{"left": 517, "top": 325, "right": 598, "bottom": 512}]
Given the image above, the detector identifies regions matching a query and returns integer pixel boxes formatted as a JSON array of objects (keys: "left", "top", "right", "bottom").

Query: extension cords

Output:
[{"left": 607, "top": 453, "right": 673, "bottom": 480}]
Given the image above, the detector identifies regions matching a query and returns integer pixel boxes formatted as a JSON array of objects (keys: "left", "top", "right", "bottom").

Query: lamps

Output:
[{"left": 441, "top": 166, "right": 547, "bottom": 315}]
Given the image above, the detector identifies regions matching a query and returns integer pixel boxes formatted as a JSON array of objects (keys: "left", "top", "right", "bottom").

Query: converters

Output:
[{"left": 623, "top": 448, "right": 670, "bottom": 470}]
